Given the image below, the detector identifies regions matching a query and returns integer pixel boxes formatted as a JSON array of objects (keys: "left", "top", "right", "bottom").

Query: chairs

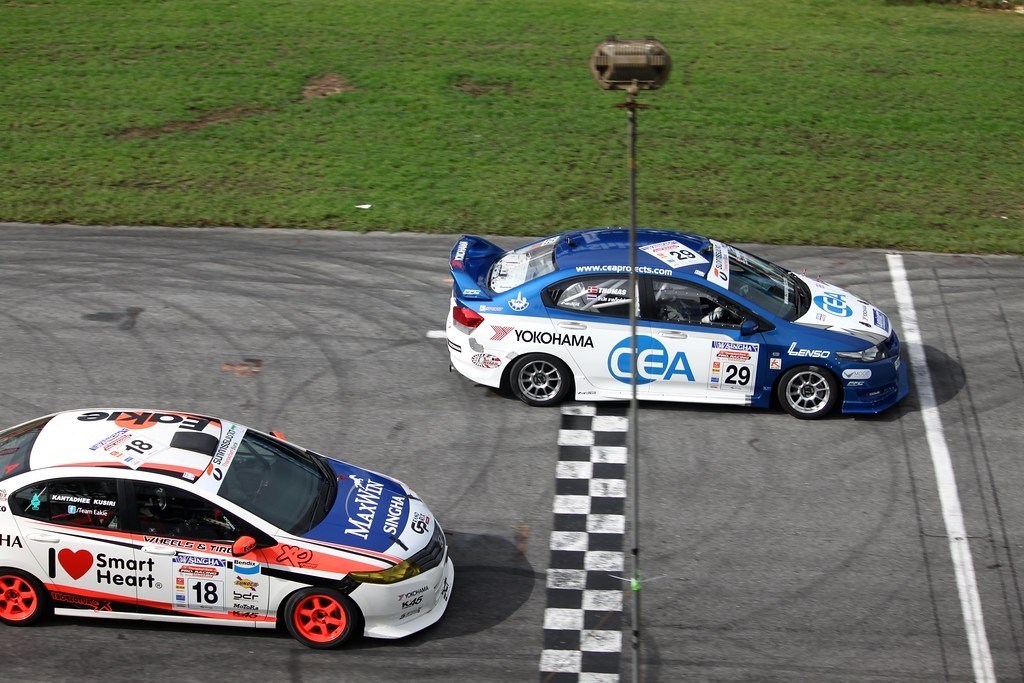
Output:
[{"left": 48, "top": 479, "right": 119, "bottom": 528}]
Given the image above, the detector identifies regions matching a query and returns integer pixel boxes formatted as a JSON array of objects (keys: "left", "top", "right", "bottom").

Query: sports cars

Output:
[
  {"left": 446, "top": 227, "right": 911, "bottom": 421},
  {"left": 0, "top": 407, "right": 454, "bottom": 650}
]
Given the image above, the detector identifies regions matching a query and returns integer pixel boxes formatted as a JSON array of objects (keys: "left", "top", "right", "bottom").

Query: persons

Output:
[{"left": 658, "top": 283, "right": 723, "bottom": 324}]
